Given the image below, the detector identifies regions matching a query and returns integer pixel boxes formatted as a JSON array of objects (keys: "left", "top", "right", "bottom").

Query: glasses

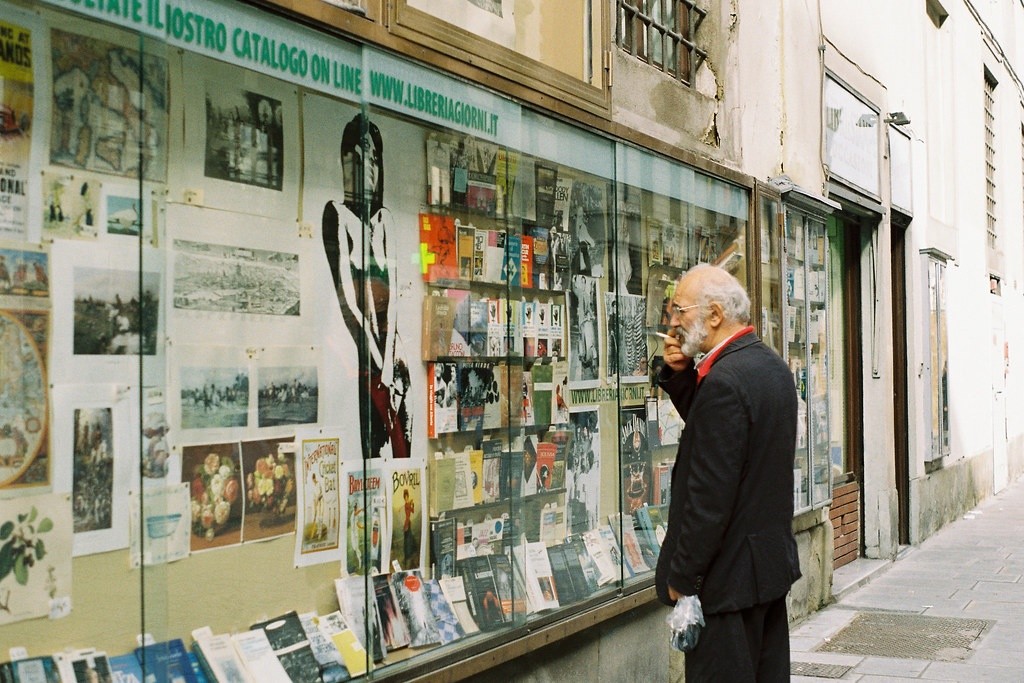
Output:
[{"left": 673, "top": 303, "right": 708, "bottom": 319}]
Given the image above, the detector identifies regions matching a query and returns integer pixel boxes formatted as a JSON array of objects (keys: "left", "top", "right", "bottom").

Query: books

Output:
[
  {"left": 420, "top": 140, "right": 573, "bottom": 292},
  {"left": 1, "top": 427, "right": 671, "bottom": 683},
  {"left": 421, "top": 296, "right": 457, "bottom": 361}
]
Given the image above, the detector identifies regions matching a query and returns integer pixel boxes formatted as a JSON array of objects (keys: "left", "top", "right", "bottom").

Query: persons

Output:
[
  {"left": 608, "top": 300, "right": 646, "bottom": 376},
  {"left": 568, "top": 419, "right": 599, "bottom": 532},
  {"left": 573, "top": 205, "right": 595, "bottom": 275},
  {"left": 650, "top": 262, "right": 803, "bottom": 683},
  {"left": 434, "top": 363, "right": 457, "bottom": 407},
  {"left": 573, "top": 276, "right": 597, "bottom": 363}
]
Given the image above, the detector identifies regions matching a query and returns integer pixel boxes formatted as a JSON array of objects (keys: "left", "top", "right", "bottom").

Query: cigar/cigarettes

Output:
[{"left": 655, "top": 332, "right": 670, "bottom": 338}]
